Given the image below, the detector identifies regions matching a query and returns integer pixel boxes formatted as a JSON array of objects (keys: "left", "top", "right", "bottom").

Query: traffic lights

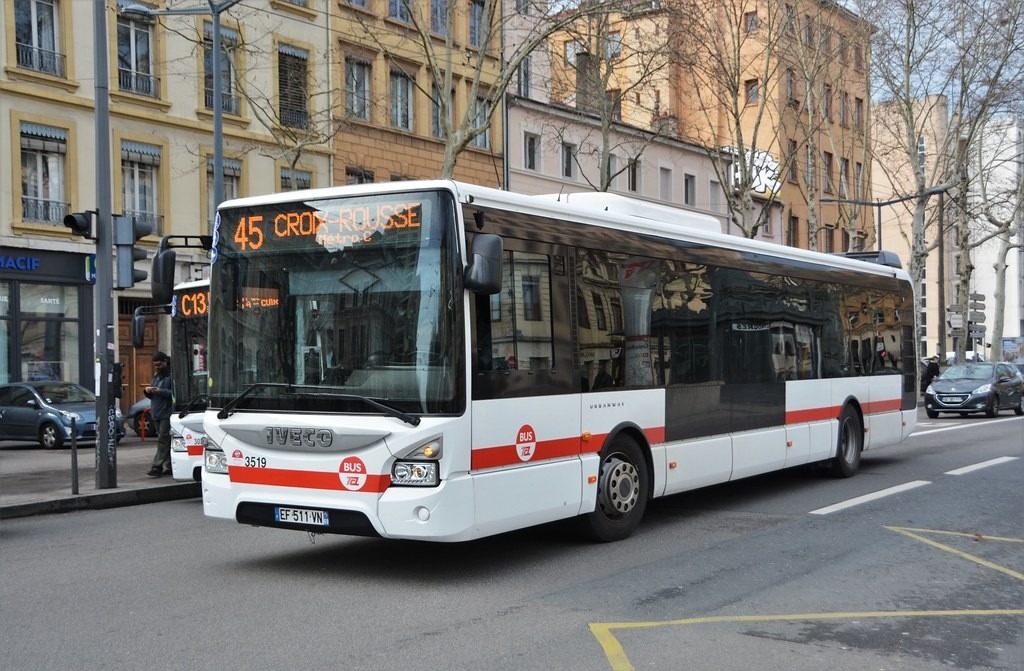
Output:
[
  {"left": 114, "top": 214, "right": 152, "bottom": 289},
  {"left": 64, "top": 212, "right": 90, "bottom": 237}
]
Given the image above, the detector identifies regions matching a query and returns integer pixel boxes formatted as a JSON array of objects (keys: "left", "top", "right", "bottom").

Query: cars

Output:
[
  {"left": 923, "top": 362, "right": 1024, "bottom": 419},
  {"left": 129, "top": 369, "right": 270, "bottom": 436},
  {"left": 0, "top": 380, "right": 128, "bottom": 450}
]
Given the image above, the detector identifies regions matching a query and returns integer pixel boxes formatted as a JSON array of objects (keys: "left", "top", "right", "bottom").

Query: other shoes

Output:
[
  {"left": 147, "top": 470, "right": 162, "bottom": 476},
  {"left": 161, "top": 470, "right": 172, "bottom": 475}
]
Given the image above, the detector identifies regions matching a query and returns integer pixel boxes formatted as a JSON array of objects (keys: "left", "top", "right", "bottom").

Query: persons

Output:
[
  {"left": 972, "top": 353, "right": 984, "bottom": 362},
  {"left": 144, "top": 352, "right": 171, "bottom": 477},
  {"left": 926, "top": 356, "right": 940, "bottom": 388},
  {"left": 590, "top": 363, "right": 614, "bottom": 389}
]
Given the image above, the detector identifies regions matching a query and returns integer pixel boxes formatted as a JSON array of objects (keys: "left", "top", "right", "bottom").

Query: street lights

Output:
[
  {"left": 120, "top": 0, "right": 240, "bottom": 204},
  {"left": 818, "top": 185, "right": 946, "bottom": 250}
]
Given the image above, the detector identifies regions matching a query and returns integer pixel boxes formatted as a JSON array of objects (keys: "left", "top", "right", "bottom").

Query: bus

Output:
[{"left": 129, "top": 179, "right": 919, "bottom": 546}]
